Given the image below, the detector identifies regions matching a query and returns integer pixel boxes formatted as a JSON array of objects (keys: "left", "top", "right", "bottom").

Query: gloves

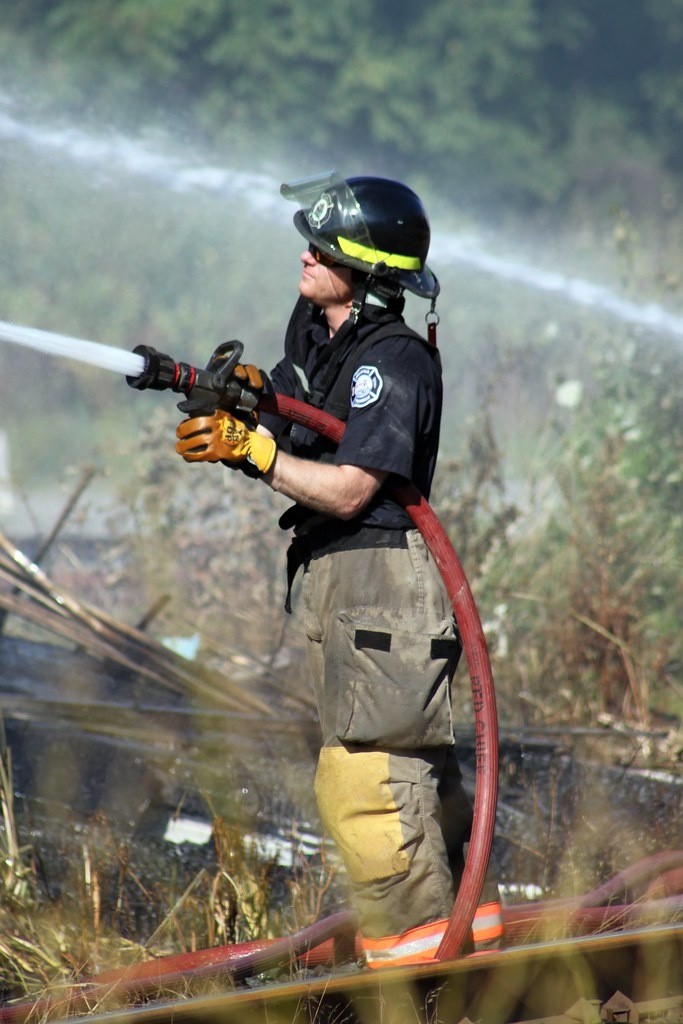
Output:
[
  {"left": 212, "top": 354, "right": 272, "bottom": 398},
  {"left": 176, "top": 404, "right": 277, "bottom": 479}
]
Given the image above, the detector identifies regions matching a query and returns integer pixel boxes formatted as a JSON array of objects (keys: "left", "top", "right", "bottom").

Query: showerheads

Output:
[{"left": 125, "top": 339, "right": 278, "bottom": 418}]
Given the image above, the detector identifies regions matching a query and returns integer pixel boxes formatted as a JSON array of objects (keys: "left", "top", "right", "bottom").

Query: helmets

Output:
[{"left": 293, "top": 176, "right": 440, "bottom": 299}]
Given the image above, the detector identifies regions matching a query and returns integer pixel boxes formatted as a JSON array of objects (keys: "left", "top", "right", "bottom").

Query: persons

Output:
[{"left": 177, "top": 176, "right": 506, "bottom": 1024}]
